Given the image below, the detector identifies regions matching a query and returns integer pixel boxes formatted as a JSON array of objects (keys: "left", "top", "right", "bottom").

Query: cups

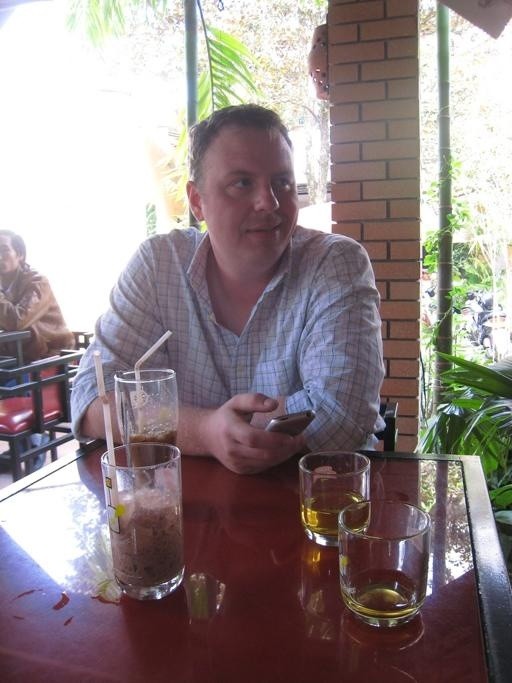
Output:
[
  {"left": 298, "top": 539, "right": 347, "bottom": 642},
  {"left": 337, "top": 499, "right": 432, "bottom": 629},
  {"left": 337, "top": 605, "right": 427, "bottom": 683},
  {"left": 101, "top": 442, "right": 188, "bottom": 600},
  {"left": 299, "top": 452, "right": 371, "bottom": 547},
  {"left": 112, "top": 367, "right": 181, "bottom": 480}
]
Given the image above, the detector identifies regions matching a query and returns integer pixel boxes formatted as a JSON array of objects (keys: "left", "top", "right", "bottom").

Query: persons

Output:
[
  {"left": 67, "top": 102, "right": 386, "bottom": 475},
  {"left": 0, "top": 230, "right": 76, "bottom": 399}
]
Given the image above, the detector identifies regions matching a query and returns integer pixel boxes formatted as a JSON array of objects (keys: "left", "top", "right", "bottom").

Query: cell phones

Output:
[{"left": 264, "top": 409, "right": 317, "bottom": 435}]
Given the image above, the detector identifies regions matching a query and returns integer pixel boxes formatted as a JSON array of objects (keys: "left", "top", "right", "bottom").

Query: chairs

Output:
[
  {"left": 373, "top": 398, "right": 404, "bottom": 450},
  {"left": 0, "top": 347, "right": 85, "bottom": 487},
  {"left": 64, "top": 329, "right": 96, "bottom": 390}
]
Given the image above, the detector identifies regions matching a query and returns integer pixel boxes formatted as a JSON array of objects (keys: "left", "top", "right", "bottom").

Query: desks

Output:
[{"left": 0, "top": 326, "right": 37, "bottom": 396}]
